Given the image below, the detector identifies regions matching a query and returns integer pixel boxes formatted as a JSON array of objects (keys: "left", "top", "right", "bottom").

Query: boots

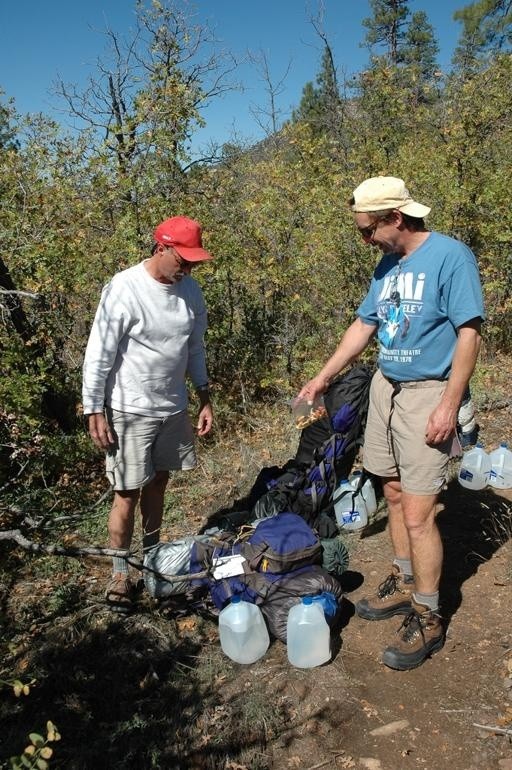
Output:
[
  {"left": 355, "top": 562, "right": 415, "bottom": 621},
  {"left": 382, "top": 593, "right": 445, "bottom": 670}
]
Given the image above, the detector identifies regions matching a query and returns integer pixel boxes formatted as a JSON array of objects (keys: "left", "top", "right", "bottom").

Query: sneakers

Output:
[{"left": 105, "top": 572, "right": 132, "bottom": 613}]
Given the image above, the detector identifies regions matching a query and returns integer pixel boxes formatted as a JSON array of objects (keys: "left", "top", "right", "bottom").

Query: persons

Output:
[
  {"left": 390, "top": 291, "right": 406, "bottom": 333},
  {"left": 291, "top": 175, "right": 487, "bottom": 671},
  {"left": 81, "top": 216, "right": 214, "bottom": 613}
]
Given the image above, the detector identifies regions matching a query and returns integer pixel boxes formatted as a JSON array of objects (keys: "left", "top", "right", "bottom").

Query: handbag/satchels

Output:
[{"left": 244, "top": 513, "right": 322, "bottom": 575}]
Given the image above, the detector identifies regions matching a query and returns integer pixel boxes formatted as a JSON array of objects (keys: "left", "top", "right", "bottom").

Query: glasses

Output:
[
  {"left": 355, "top": 212, "right": 394, "bottom": 239},
  {"left": 166, "top": 246, "right": 198, "bottom": 271}
]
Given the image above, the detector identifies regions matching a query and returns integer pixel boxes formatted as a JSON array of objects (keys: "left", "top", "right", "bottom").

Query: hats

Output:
[
  {"left": 154, "top": 215, "right": 213, "bottom": 262},
  {"left": 352, "top": 175, "right": 432, "bottom": 220}
]
